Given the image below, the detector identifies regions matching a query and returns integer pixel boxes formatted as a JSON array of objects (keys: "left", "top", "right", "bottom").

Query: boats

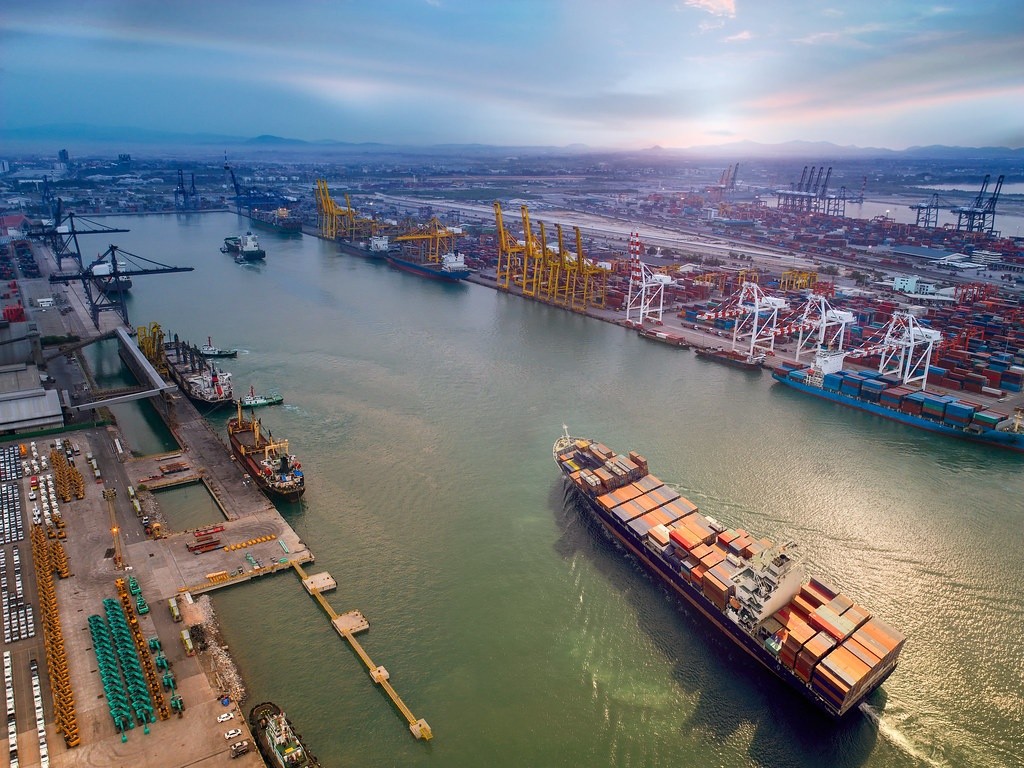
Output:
[
  {"left": 638, "top": 327, "right": 690, "bottom": 349},
  {"left": 227, "top": 401, "right": 306, "bottom": 502},
  {"left": 693, "top": 344, "right": 766, "bottom": 371},
  {"left": 248, "top": 702, "right": 324, "bottom": 768},
  {"left": 249, "top": 208, "right": 303, "bottom": 234},
  {"left": 772, "top": 360, "right": 1024, "bottom": 454},
  {"left": 224, "top": 231, "right": 266, "bottom": 260},
  {"left": 88, "top": 260, "right": 133, "bottom": 291},
  {"left": 551, "top": 420, "right": 907, "bottom": 722},
  {"left": 232, "top": 384, "right": 284, "bottom": 408},
  {"left": 385, "top": 248, "right": 470, "bottom": 284},
  {"left": 159, "top": 330, "right": 234, "bottom": 408},
  {"left": 197, "top": 335, "right": 238, "bottom": 358}
]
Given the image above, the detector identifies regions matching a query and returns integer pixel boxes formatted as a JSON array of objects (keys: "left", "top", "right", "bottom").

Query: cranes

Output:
[
  {"left": 625, "top": 227, "right": 664, "bottom": 324},
  {"left": 704, "top": 162, "right": 741, "bottom": 203},
  {"left": 493, "top": 200, "right": 605, "bottom": 310},
  {"left": 47, "top": 243, "right": 195, "bottom": 327},
  {"left": 172, "top": 170, "right": 202, "bottom": 210},
  {"left": 772, "top": 164, "right": 865, "bottom": 218},
  {"left": 908, "top": 174, "right": 1005, "bottom": 239},
  {"left": 696, "top": 279, "right": 942, "bottom": 390},
  {"left": 223, "top": 165, "right": 298, "bottom": 216},
  {"left": 25, "top": 175, "right": 130, "bottom": 267},
  {"left": 312, "top": 179, "right": 468, "bottom": 262}
]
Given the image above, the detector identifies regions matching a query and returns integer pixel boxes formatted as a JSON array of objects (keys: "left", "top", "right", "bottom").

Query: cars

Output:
[
  {"left": 230, "top": 741, "right": 248, "bottom": 750},
  {"left": 230, "top": 746, "right": 248, "bottom": 759},
  {"left": 225, "top": 728, "right": 243, "bottom": 739},
  {"left": 216, "top": 713, "right": 234, "bottom": 723}
]
